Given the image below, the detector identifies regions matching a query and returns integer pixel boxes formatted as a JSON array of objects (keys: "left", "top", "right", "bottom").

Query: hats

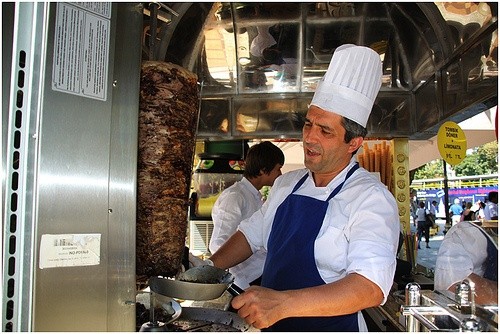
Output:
[{"left": 308, "top": 43, "right": 383, "bottom": 128}]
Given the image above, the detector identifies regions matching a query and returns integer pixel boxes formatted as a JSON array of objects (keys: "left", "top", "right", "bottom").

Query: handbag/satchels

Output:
[{"left": 424, "top": 208, "right": 433, "bottom": 226}]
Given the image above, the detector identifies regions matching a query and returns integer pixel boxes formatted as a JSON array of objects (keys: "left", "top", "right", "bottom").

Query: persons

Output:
[
  {"left": 208, "top": 139, "right": 285, "bottom": 290},
  {"left": 188, "top": 87, "right": 399, "bottom": 332},
  {"left": 412, "top": 191, "right": 498, "bottom": 251},
  {"left": 432, "top": 217, "right": 498, "bottom": 322}
]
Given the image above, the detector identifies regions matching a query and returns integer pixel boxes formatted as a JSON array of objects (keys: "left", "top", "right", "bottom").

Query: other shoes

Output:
[
  {"left": 418, "top": 245, "right": 421, "bottom": 249},
  {"left": 426, "top": 246, "right": 431, "bottom": 249},
  {"left": 414, "top": 224, "right": 416, "bottom": 226}
]
